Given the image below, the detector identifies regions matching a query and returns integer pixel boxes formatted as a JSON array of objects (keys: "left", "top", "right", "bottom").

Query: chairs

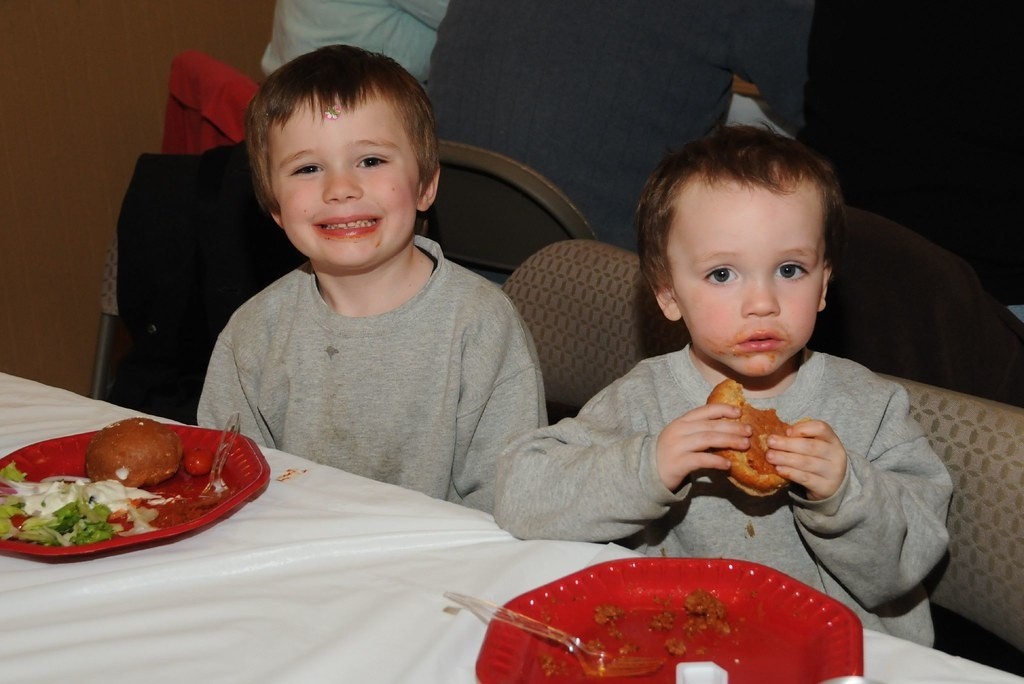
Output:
[
  {"left": 809, "top": 199, "right": 1024, "bottom": 405},
  {"left": 853, "top": 370, "right": 1024, "bottom": 649},
  {"left": 86, "top": 46, "right": 690, "bottom": 422}
]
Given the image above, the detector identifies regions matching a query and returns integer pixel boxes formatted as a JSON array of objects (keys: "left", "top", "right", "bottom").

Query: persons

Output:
[
  {"left": 196, "top": 45, "right": 548, "bottom": 514},
  {"left": 260, "top": 0, "right": 820, "bottom": 256},
  {"left": 493, "top": 127, "right": 952, "bottom": 648}
]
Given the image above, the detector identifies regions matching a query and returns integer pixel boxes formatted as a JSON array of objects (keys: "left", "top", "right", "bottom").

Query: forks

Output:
[
  {"left": 442, "top": 591, "right": 664, "bottom": 675},
  {"left": 186, "top": 412, "right": 240, "bottom": 506}
]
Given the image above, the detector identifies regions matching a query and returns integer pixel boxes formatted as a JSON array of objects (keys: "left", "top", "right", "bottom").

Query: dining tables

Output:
[{"left": 0, "top": 369, "right": 1024, "bottom": 684}]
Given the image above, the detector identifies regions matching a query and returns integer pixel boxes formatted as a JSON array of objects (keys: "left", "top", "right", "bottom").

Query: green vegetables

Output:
[{"left": 0, "top": 462, "right": 163, "bottom": 548}]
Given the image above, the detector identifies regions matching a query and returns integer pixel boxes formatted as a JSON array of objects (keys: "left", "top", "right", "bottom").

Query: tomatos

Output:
[{"left": 183, "top": 447, "right": 214, "bottom": 475}]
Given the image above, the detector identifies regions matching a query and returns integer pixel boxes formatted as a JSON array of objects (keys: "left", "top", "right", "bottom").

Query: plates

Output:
[
  {"left": 476, "top": 556, "right": 863, "bottom": 684},
  {"left": 0, "top": 424, "right": 271, "bottom": 556}
]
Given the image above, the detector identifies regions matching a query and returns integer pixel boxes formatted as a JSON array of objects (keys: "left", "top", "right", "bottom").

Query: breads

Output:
[
  {"left": 84, "top": 417, "right": 182, "bottom": 488},
  {"left": 703, "top": 379, "right": 815, "bottom": 496}
]
